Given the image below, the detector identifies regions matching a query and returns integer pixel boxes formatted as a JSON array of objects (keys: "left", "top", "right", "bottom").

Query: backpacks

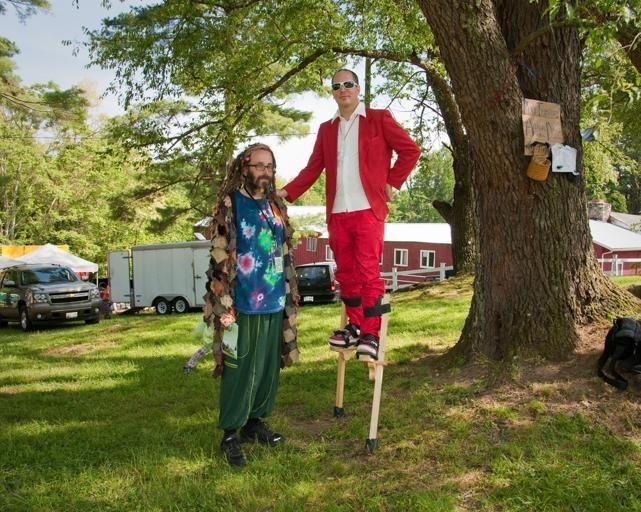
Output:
[{"left": 597, "top": 318, "right": 641, "bottom": 390}]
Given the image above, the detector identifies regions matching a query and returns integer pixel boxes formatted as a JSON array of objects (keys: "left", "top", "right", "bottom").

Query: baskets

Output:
[{"left": 527, "top": 144, "right": 551, "bottom": 181}]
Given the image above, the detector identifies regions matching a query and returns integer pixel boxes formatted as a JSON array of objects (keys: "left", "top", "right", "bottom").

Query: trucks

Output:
[{"left": 105, "top": 240, "right": 212, "bottom": 314}]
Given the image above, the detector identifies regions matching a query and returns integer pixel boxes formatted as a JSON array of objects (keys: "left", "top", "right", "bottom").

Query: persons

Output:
[
  {"left": 275, "top": 67, "right": 422, "bottom": 361},
  {"left": 200, "top": 141, "right": 300, "bottom": 470}
]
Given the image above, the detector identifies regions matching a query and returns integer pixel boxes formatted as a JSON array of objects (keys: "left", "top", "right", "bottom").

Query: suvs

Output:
[
  {"left": 293, "top": 261, "right": 339, "bottom": 303},
  {"left": 1, "top": 262, "right": 102, "bottom": 332}
]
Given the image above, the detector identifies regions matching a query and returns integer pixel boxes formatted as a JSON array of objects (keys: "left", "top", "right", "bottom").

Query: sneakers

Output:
[
  {"left": 356, "top": 335, "right": 378, "bottom": 360},
  {"left": 329, "top": 324, "right": 361, "bottom": 346},
  {"left": 221, "top": 432, "right": 245, "bottom": 466},
  {"left": 240, "top": 419, "right": 284, "bottom": 446}
]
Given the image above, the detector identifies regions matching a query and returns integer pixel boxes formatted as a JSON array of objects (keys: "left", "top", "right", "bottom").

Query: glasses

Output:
[
  {"left": 332, "top": 81, "right": 358, "bottom": 90},
  {"left": 246, "top": 163, "right": 273, "bottom": 172}
]
